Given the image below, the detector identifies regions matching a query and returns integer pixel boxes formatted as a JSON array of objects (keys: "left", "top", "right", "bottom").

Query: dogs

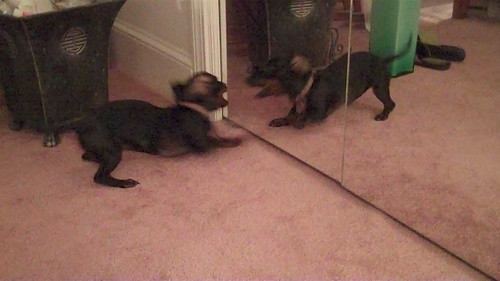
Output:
[
  {"left": 69, "top": 71, "right": 242, "bottom": 189},
  {"left": 246, "top": 32, "right": 413, "bottom": 129}
]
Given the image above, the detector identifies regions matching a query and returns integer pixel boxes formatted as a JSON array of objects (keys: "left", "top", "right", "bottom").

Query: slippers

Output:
[
  {"left": 416, "top": 42, "right": 450, "bottom": 69},
  {"left": 425, "top": 42, "right": 466, "bottom": 62}
]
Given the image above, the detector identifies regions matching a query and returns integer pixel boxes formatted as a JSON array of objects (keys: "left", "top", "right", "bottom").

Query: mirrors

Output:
[{"left": 226, "top": 1, "right": 499, "bottom": 280}]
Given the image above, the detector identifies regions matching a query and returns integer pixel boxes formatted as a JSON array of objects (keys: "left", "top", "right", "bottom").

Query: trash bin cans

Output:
[
  {"left": 0, "top": 0, "right": 128, "bottom": 147},
  {"left": 239, "top": 0, "right": 338, "bottom": 87}
]
